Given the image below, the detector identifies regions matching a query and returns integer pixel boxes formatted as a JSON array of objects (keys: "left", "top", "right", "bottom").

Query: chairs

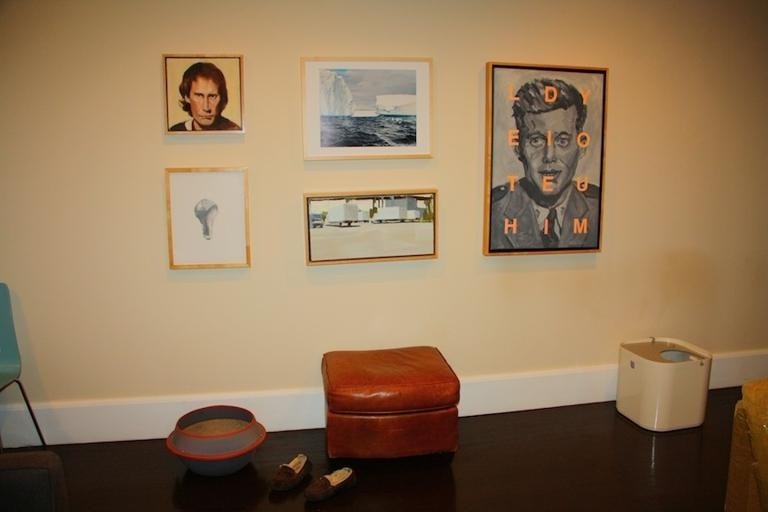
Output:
[{"left": 0, "top": 281, "right": 50, "bottom": 453}]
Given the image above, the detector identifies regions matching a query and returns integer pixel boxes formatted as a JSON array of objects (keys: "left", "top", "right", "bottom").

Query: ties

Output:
[{"left": 540, "top": 208, "right": 560, "bottom": 248}]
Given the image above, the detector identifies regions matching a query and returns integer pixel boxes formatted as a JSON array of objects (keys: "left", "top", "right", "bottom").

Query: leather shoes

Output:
[
  {"left": 268, "top": 452, "right": 313, "bottom": 494},
  {"left": 303, "top": 464, "right": 353, "bottom": 502}
]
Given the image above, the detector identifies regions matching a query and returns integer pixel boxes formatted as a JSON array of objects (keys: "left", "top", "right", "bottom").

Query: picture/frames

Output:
[
  {"left": 165, "top": 167, "right": 253, "bottom": 270},
  {"left": 162, "top": 52, "right": 247, "bottom": 136},
  {"left": 482, "top": 62, "right": 612, "bottom": 256},
  {"left": 299, "top": 56, "right": 437, "bottom": 163},
  {"left": 303, "top": 188, "right": 441, "bottom": 267}
]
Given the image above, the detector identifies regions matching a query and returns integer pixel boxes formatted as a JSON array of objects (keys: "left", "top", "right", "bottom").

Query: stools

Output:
[{"left": 321, "top": 345, "right": 462, "bottom": 460}]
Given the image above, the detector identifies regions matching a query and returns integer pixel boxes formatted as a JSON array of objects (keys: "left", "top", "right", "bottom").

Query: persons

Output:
[
  {"left": 169, "top": 62, "right": 242, "bottom": 132},
  {"left": 491, "top": 77, "right": 601, "bottom": 250}
]
